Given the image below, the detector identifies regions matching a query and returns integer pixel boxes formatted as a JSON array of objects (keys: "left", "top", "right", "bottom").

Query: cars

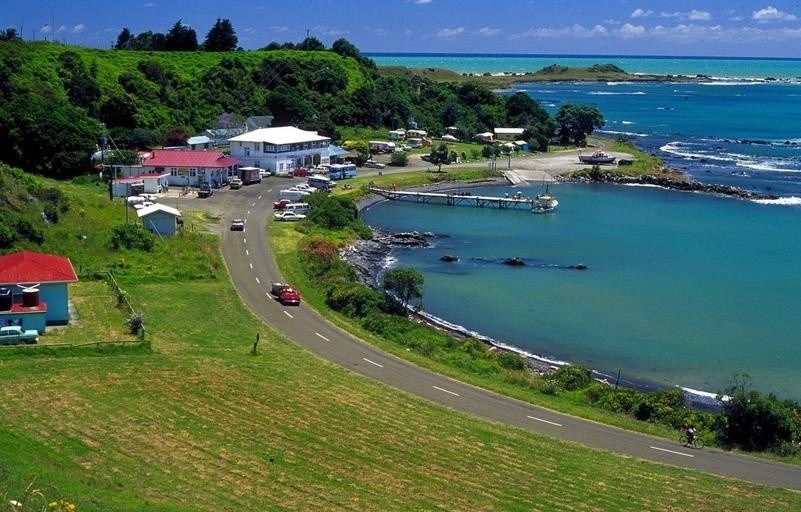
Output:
[
  {"left": 231, "top": 218, "right": 244, "bottom": 231},
  {"left": 272, "top": 183, "right": 318, "bottom": 221},
  {"left": 230, "top": 178, "right": 244, "bottom": 189},
  {"left": 442, "top": 135, "right": 461, "bottom": 142},
  {"left": 279, "top": 283, "right": 301, "bottom": 305},
  {"left": 0, "top": 325, "right": 39, "bottom": 344}
]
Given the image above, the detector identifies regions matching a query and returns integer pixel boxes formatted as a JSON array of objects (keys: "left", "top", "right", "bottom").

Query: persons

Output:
[
  {"left": 686, "top": 425, "right": 696, "bottom": 445},
  {"left": 513, "top": 189, "right": 530, "bottom": 200},
  {"left": 391, "top": 183, "right": 396, "bottom": 190},
  {"left": 505, "top": 191, "right": 509, "bottom": 197}
]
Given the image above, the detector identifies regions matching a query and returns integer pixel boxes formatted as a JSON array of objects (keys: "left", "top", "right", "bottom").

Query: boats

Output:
[{"left": 579, "top": 151, "right": 616, "bottom": 165}]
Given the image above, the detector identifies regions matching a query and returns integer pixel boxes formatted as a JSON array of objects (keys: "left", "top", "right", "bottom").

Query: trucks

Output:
[
  {"left": 198, "top": 183, "right": 213, "bottom": 198},
  {"left": 239, "top": 167, "right": 262, "bottom": 186}
]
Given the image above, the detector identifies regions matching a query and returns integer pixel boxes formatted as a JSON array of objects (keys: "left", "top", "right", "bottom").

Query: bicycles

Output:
[{"left": 679, "top": 436, "right": 705, "bottom": 449}]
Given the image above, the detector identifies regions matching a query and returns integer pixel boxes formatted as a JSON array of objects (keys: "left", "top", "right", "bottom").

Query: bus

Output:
[
  {"left": 308, "top": 175, "right": 333, "bottom": 189},
  {"left": 329, "top": 163, "right": 357, "bottom": 180},
  {"left": 368, "top": 140, "right": 395, "bottom": 153}
]
